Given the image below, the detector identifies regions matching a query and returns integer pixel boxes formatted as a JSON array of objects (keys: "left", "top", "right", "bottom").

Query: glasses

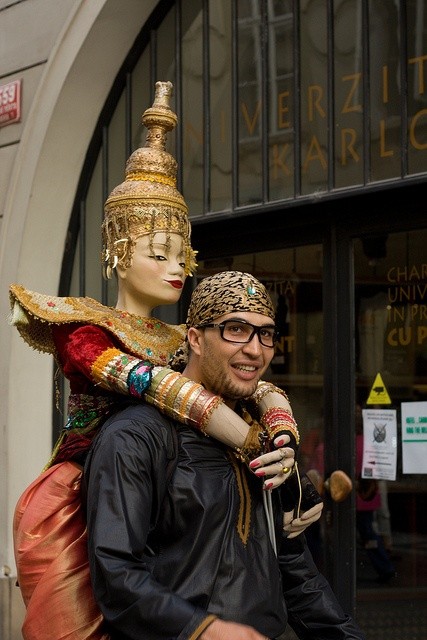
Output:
[{"left": 196, "top": 320, "right": 277, "bottom": 348}]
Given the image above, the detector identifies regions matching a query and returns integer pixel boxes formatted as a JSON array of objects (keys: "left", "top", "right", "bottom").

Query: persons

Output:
[
  {"left": 10, "top": 81, "right": 297, "bottom": 640},
  {"left": 81, "top": 271, "right": 369, "bottom": 640}
]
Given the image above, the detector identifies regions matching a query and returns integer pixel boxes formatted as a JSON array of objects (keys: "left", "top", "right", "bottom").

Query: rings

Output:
[{"left": 278, "top": 448, "right": 290, "bottom": 474}]
